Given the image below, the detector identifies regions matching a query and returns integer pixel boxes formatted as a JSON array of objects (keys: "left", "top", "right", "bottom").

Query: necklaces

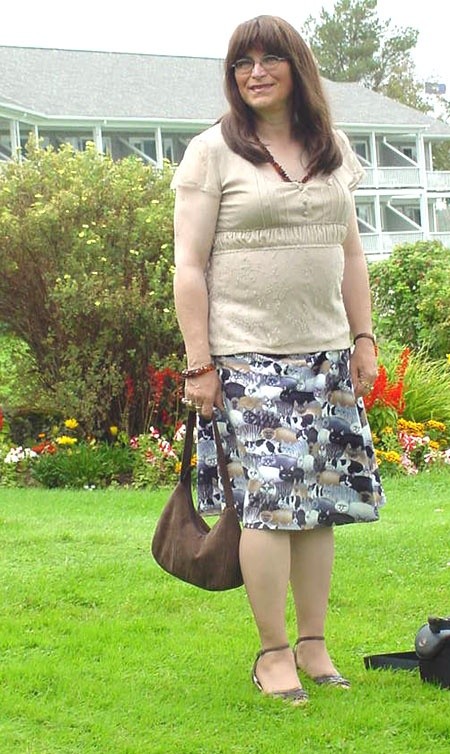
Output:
[{"left": 255, "top": 133, "right": 312, "bottom": 183}]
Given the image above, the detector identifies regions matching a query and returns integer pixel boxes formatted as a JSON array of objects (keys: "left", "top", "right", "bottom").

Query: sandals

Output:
[
  {"left": 295, "top": 635, "right": 357, "bottom": 690},
  {"left": 250, "top": 643, "right": 311, "bottom": 705}
]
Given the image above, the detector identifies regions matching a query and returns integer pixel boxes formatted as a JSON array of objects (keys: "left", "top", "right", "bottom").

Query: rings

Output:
[
  {"left": 360, "top": 380, "right": 373, "bottom": 390},
  {"left": 182, "top": 398, "right": 201, "bottom": 409}
]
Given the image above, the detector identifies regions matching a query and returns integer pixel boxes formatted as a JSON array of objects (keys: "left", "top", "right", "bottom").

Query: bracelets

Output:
[
  {"left": 354, "top": 333, "right": 376, "bottom": 345},
  {"left": 181, "top": 362, "right": 215, "bottom": 378}
]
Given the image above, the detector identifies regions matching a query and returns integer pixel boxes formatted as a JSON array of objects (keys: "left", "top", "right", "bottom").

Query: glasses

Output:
[{"left": 230, "top": 54, "right": 289, "bottom": 73}]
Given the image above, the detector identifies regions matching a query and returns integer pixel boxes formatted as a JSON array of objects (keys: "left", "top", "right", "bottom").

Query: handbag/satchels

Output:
[
  {"left": 148, "top": 402, "right": 253, "bottom": 592},
  {"left": 363, "top": 617, "right": 450, "bottom": 687}
]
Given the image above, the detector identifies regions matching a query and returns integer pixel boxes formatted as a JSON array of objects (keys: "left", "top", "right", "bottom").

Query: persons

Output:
[{"left": 171, "top": 15, "right": 378, "bottom": 705}]
